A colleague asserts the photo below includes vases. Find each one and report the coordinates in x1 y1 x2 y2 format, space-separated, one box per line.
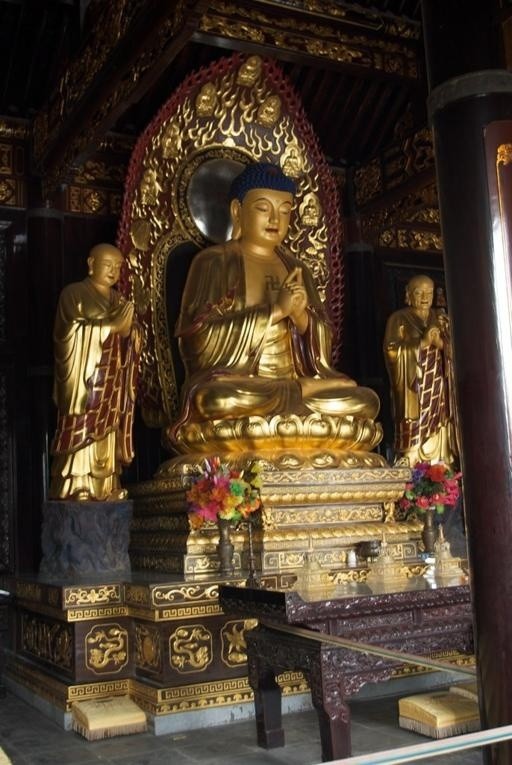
420 506 439 553
215 517 235 573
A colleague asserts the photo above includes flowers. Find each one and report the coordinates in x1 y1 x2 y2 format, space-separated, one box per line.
185 454 265 528
394 462 463 523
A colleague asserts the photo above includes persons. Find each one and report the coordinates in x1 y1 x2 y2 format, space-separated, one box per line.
173 159 386 452
46 243 149 504
382 273 462 471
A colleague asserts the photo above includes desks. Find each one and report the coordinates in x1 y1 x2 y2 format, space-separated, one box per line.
218 558 474 762
